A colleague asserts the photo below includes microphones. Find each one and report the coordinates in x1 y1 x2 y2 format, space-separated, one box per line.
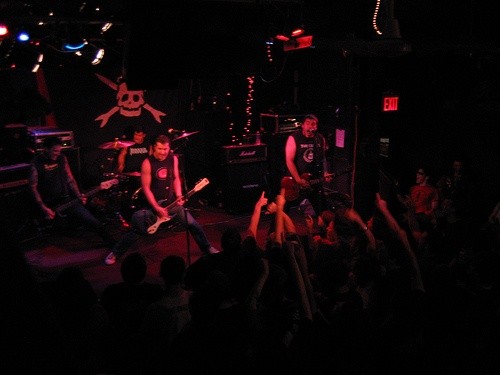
310 128 319 138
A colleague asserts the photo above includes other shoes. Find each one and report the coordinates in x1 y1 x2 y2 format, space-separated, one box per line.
105 251 116 265
201 246 220 254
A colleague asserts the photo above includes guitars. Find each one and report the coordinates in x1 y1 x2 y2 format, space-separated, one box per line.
281 164 359 202
141 177 211 236
34 178 120 237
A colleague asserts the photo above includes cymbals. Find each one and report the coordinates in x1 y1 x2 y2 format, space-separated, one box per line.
97 140 136 150
121 170 141 177
170 129 200 142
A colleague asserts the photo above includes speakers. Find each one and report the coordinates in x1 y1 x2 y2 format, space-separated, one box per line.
0 188 44 243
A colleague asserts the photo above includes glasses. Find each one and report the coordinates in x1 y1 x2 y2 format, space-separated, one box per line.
417 169 428 176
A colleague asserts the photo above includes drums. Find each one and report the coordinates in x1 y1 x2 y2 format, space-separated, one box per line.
130 186 180 231
102 172 128 196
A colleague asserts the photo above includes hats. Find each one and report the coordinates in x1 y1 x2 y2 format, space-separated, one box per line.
305 113 318 122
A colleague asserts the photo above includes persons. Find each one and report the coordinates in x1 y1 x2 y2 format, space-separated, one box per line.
284 113 331 216
15 136 118 248
104 135 221 266
397 166 440 223
0 188 500 375
